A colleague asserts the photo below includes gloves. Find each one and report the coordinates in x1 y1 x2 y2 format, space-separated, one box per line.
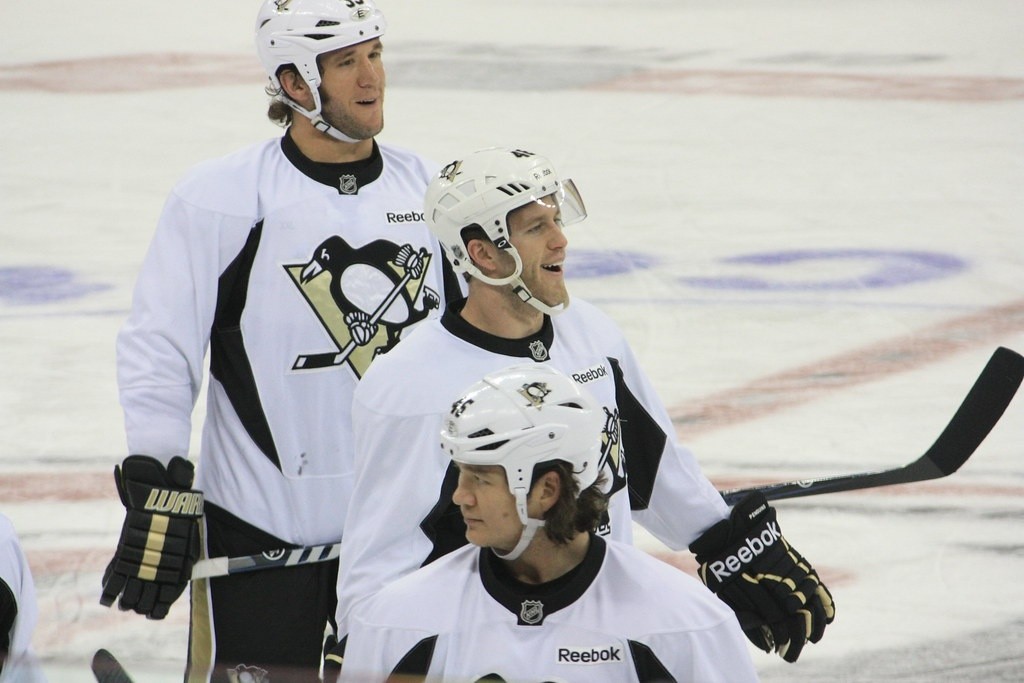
693 492 833 659
101 460 206 616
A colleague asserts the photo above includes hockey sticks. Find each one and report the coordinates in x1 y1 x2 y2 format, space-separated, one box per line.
187 345 1024 585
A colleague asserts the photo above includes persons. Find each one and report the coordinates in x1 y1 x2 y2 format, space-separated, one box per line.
327 365 759 682
0 510 44 683
324 148 839 682
98 2 456 682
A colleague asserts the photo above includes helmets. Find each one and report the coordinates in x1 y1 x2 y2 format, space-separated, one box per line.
256 0 386 142
422 146 587 316
441 361 612 563
